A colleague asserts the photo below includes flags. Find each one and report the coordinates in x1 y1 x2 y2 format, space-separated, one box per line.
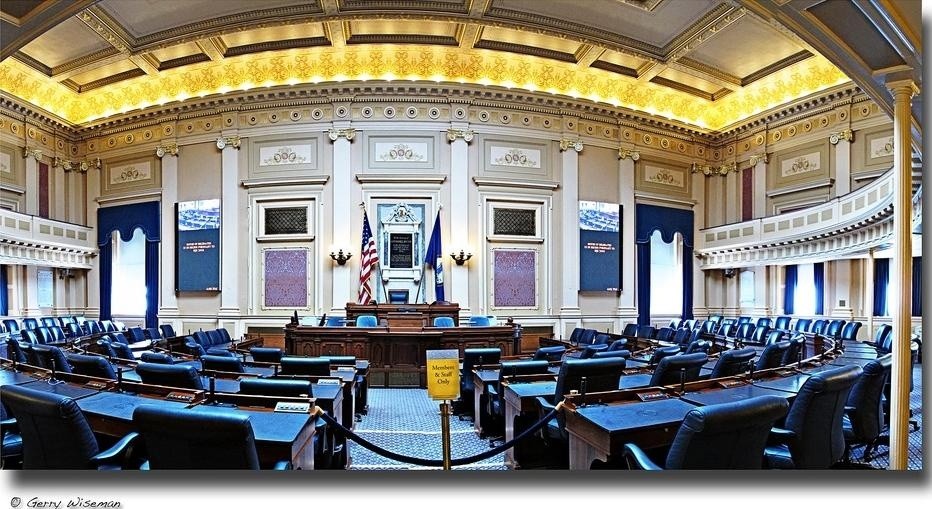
356 206 382 305
426 212 449 303
